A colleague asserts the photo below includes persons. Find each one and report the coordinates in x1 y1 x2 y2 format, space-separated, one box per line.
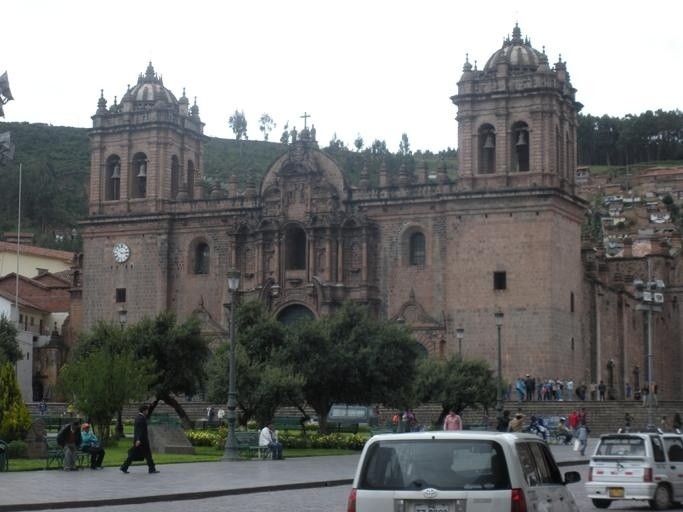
557 407 592 456
669 444 683 462
434 402 463 431
393 408 418 433
575 380 606 401
79 423 105 470
504 375 576 403
56 420 83 471
258 423 285 460
217 407 225 421
617 410 683 434
625 382 659 408
38 399 47 415
496 407 547 440
206 405 216 421
120 405 160 475
67 402 74 417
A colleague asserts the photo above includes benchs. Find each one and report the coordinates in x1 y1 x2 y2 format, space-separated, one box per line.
542 426 570 444
234 431 273 460
43 437 92 469
31 415 81 433
271 416 306 436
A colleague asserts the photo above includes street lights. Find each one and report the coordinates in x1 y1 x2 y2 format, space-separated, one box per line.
633 278 665 430
221 265 244 459
607 359 617 400
631 365 642 401
455 323 464 357
492 309 504 420
0 68 18 162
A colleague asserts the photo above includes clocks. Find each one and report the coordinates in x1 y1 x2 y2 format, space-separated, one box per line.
113 243 131 264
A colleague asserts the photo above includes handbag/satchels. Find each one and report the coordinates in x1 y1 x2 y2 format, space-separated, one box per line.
128 442 145 461
91 441 100 448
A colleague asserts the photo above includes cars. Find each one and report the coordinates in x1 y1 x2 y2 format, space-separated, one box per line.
585 428 682 508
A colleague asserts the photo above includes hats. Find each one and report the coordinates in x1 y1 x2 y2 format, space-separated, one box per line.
82 424 90 429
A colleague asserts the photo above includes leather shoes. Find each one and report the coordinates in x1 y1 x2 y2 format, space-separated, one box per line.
149 470 160 473
120 468 129 473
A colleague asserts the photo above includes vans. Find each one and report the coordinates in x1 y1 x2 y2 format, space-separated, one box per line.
346 431 582 512
327 404 372 426
